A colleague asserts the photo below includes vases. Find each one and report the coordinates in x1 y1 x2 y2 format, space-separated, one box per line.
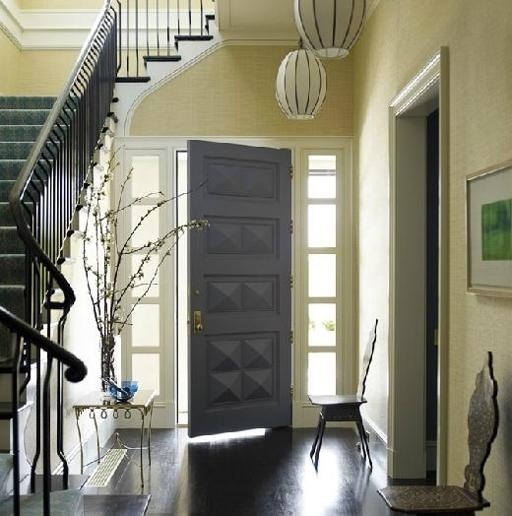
97 339 117 399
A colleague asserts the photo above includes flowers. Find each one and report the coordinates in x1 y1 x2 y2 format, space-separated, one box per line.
81 145 208 393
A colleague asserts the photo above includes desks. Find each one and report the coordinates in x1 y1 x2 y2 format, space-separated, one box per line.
71 392 155 488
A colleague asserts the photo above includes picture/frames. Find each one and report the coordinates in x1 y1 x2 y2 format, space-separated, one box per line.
463 160 512 299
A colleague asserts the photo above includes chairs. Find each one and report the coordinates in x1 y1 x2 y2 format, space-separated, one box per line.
308 319 379 472
377 350 498 516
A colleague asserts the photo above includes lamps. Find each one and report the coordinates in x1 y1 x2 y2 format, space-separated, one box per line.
293 0 368 59
274 36 328 121
